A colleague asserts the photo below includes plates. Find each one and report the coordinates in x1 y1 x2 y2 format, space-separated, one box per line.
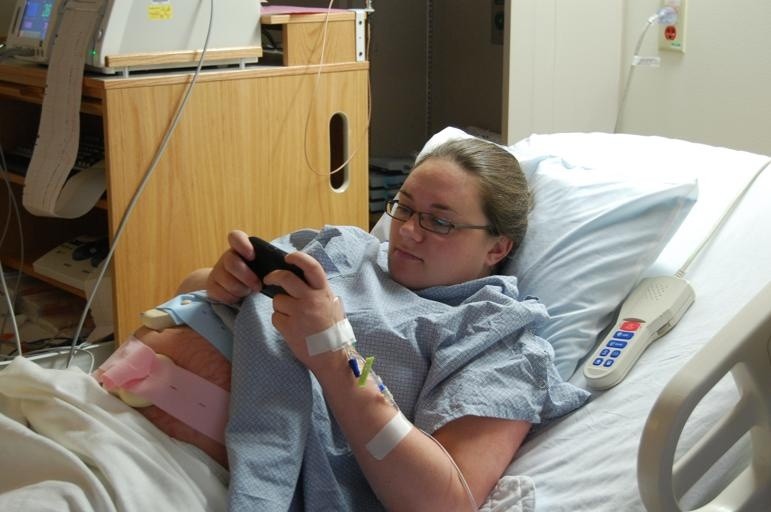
1 10 370 349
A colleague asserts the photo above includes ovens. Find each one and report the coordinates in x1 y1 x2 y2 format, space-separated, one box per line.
0 135 771 511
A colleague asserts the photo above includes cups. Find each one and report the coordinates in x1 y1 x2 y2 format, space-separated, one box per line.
385 199 488 234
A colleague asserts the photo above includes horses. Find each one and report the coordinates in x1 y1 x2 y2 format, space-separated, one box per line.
244 237 304 297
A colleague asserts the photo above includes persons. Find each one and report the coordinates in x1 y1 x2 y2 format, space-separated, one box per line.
0 138 591 512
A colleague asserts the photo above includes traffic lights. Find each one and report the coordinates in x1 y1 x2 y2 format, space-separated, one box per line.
379 127 771 380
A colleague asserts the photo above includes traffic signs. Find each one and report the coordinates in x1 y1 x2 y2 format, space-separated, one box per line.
658 0 686 52
